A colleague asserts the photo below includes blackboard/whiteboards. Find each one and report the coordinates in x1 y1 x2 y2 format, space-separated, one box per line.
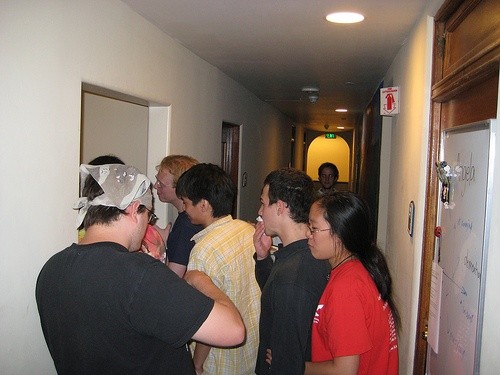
424 118 494 375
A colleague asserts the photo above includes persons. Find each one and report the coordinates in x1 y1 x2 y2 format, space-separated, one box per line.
312 162 339 198
175 163 261 375
266 191 402 375
35 155 246 375
154 153 205 279
253 167 332 375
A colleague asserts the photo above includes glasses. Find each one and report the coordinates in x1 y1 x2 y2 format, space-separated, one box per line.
145 206 159 225
307 225 331 235
154 176 176 188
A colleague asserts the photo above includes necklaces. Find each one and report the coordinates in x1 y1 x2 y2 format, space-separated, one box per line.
325 254 354 281
